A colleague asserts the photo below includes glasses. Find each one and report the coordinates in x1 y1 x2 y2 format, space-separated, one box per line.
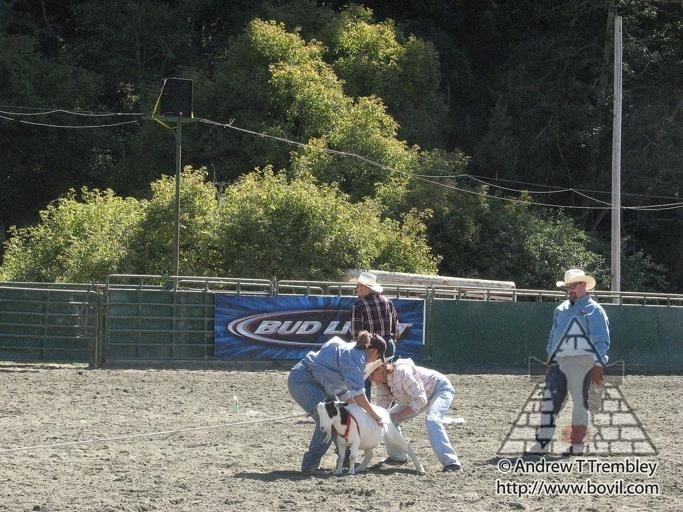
564 282 583 291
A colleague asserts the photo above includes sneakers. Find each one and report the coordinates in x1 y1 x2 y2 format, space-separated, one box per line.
569 443 584 454
525 439 549 453
303 469 333 479
378 457 410 468
442 464 463 476
337 460 361 469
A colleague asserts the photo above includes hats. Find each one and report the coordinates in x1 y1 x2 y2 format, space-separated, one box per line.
363 355 395 381
373 333 386 364
348 272 384 293
555 269 595 291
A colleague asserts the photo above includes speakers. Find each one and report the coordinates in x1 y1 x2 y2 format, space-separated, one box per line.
161 78 193 121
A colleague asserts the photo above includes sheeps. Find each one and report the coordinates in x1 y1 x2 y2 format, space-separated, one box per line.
306 400 425 475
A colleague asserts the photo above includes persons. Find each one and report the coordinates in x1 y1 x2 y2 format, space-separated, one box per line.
363 357 460 473
349 271 400 403
287 329 386 477
527 268 609 455
